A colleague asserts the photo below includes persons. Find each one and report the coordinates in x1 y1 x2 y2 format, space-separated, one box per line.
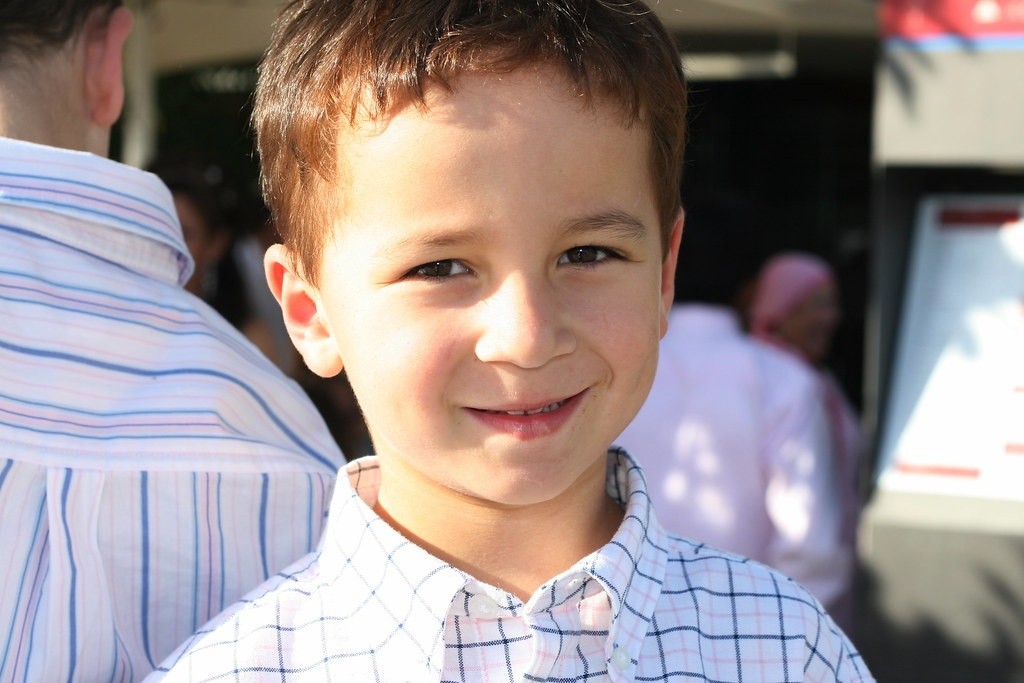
146 0 876 683
0 1 877 683
